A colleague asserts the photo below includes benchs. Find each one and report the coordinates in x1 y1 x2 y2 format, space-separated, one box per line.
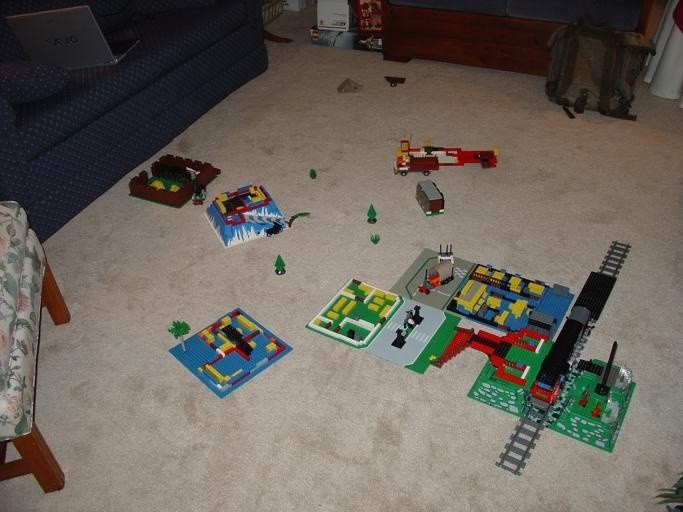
379 1 666 88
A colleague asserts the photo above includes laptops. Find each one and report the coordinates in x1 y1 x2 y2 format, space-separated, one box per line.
6 5 143 72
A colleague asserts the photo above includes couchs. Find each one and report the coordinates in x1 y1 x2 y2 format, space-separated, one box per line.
0 0 268 246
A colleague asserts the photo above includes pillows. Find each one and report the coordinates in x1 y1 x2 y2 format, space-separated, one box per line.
0 58 75 103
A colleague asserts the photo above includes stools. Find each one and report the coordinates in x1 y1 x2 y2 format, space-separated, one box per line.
261 0 295 44
0 199 72 493
